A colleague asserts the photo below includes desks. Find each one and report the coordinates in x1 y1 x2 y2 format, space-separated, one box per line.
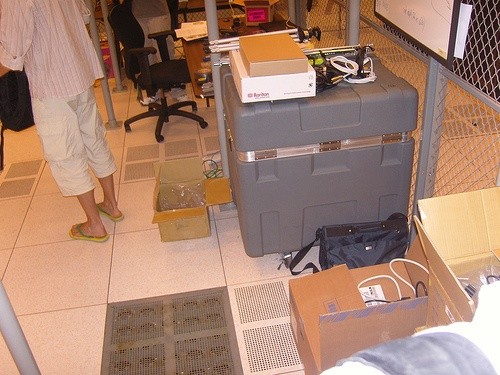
181 23 286 98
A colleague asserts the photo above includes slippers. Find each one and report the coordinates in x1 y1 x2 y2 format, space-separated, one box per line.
69 222 109 242
95 202 124 222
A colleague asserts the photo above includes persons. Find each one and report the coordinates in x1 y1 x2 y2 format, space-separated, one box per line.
131 0 187 105
315 281 500 375
0 0 125 242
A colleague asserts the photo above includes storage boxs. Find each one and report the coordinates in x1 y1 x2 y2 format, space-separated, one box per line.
151 157 231 243
229 49 317 104
239 33 308 76
220 51 419 258
231 0 279 27
414 186 500 322
288 236 428 375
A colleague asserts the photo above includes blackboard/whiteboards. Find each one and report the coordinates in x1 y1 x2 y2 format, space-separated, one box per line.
374 0 461 70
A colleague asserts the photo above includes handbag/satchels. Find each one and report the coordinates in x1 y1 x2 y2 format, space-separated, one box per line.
316 212 410 270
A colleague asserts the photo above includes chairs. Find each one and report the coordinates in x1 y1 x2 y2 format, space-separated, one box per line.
107 2 208 142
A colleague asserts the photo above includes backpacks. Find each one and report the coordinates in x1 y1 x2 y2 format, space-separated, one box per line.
0 68 34 131
